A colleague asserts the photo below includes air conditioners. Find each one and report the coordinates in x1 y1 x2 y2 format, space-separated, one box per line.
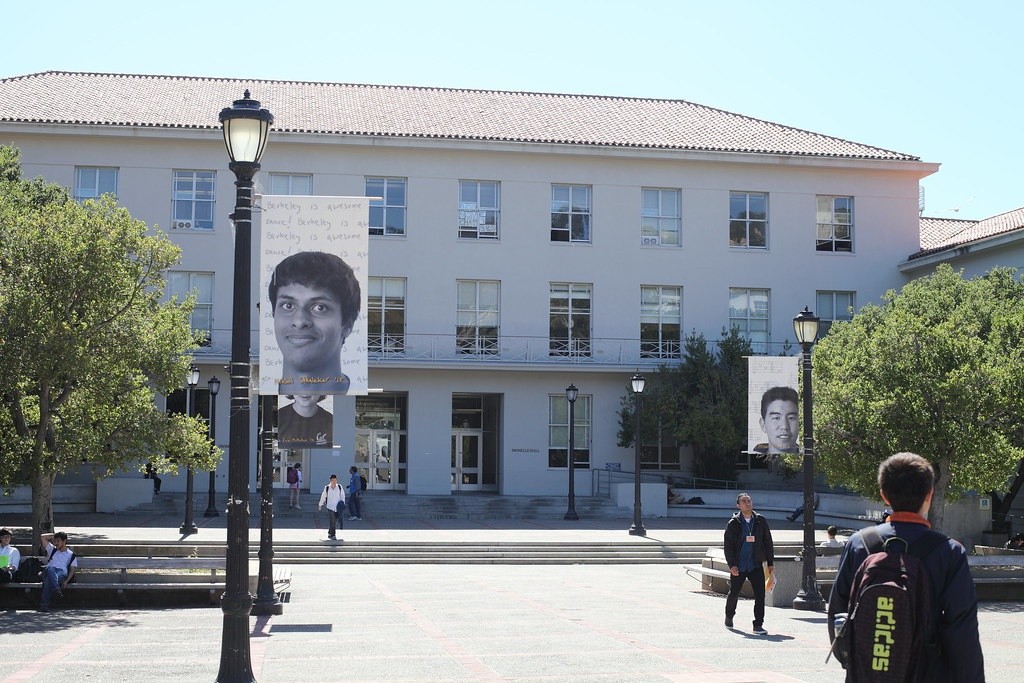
176 220 194 230
640 236 658 246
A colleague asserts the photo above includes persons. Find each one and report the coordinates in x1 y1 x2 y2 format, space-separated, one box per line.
288 462 303 509
36 533 78 612
667 482 677 504
815 525 846 569
317 474 345 541
753 387 798 454
875 512 891 525
723 493 775 634
786 489 820 522
828 451 986 683
272 452 281 482
0 528 21 585
143 463 162 495
346 466 362 521
260 251 366 395
278 395 333 449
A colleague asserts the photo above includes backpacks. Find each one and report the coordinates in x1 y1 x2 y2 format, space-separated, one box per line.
825 527 950 682
18 558 44 583
286 467 298 484
360 474 367 491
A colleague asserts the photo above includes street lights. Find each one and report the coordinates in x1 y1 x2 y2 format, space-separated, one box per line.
203 376 221 517
215 89 274 682
790 305 827 612
628 368 647 536
564 383 579 519
178 365 201 534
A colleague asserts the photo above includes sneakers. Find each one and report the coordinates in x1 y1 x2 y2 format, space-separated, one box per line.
349 516 357 520
328 534 331 538
753 625 768 633
725 616 733 629
330 536 337 541
357 518 362 520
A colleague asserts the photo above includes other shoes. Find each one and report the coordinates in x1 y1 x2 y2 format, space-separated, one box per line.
295 506 301 509
55 590 64 601
37 606 46 612
289 505 292 509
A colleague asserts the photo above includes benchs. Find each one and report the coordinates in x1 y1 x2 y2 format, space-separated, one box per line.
666 487 895 530
0 544 228 605
